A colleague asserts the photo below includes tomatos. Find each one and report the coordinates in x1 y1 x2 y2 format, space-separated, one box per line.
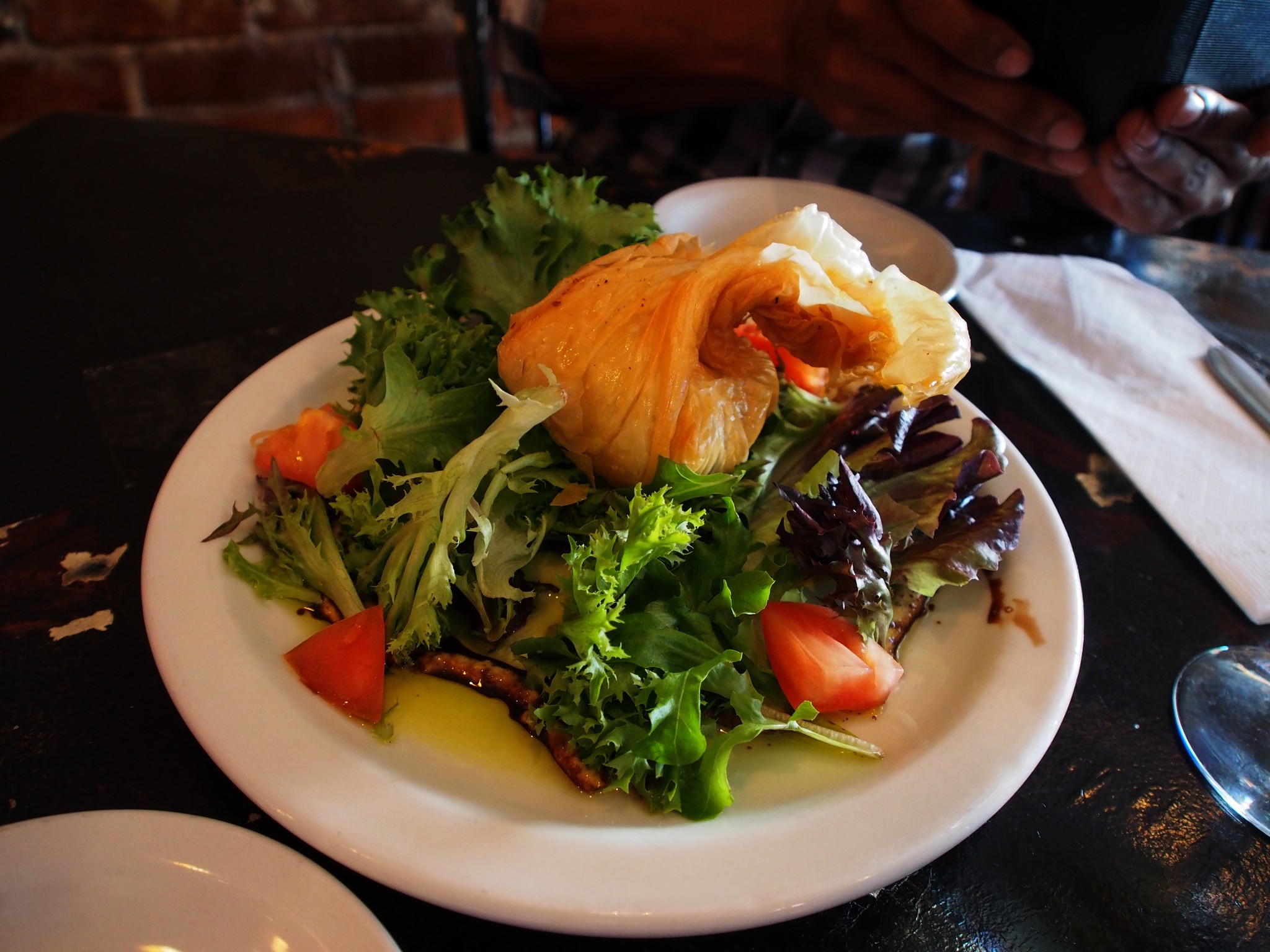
759 598 902 716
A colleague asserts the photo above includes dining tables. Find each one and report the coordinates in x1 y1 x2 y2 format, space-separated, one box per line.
1 106 1270 951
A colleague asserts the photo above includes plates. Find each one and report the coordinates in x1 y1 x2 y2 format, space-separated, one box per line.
142 292 1083 936
0 809 404 952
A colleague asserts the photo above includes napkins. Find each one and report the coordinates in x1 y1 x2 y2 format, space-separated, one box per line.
948 249 1270 626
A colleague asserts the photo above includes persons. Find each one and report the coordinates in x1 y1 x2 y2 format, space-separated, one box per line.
497 0 1269 241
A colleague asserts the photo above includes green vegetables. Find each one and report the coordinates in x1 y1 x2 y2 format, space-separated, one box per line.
203 163 884 821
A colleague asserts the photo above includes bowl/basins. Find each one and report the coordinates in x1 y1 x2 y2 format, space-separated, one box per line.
651 176 960 302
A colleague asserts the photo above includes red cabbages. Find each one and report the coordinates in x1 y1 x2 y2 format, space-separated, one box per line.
746 380 1025 632
